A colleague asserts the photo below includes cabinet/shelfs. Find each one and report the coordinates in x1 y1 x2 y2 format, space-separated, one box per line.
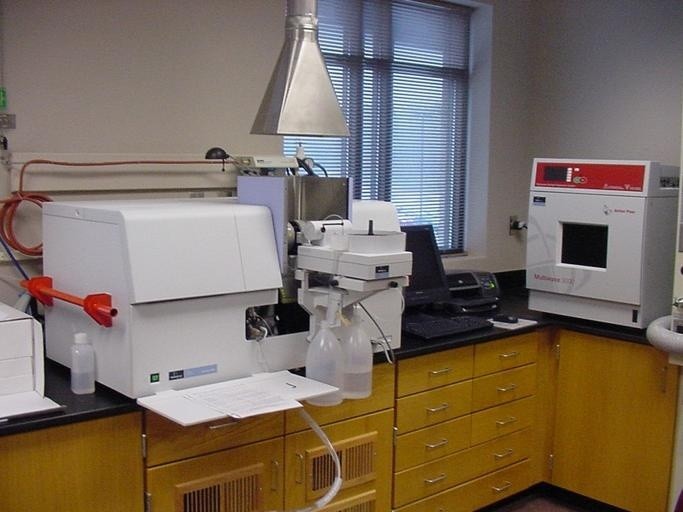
549 329 678 512
395 326 539 512
0 410 145 512
144 362 395 512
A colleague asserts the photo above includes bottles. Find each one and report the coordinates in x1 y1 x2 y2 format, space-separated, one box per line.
71 333 94 394
306 321 344 407
342 316 372 398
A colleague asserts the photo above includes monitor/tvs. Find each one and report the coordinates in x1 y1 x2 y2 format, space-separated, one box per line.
400 224 451 308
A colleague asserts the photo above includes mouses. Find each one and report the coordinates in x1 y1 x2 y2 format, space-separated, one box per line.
493 313 518 324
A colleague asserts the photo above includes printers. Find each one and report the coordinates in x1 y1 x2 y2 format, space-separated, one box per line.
446 268 503 316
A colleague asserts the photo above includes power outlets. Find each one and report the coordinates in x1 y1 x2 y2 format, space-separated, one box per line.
510 215 518 235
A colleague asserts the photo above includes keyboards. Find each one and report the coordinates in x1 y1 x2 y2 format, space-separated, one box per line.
402 313 494 341
